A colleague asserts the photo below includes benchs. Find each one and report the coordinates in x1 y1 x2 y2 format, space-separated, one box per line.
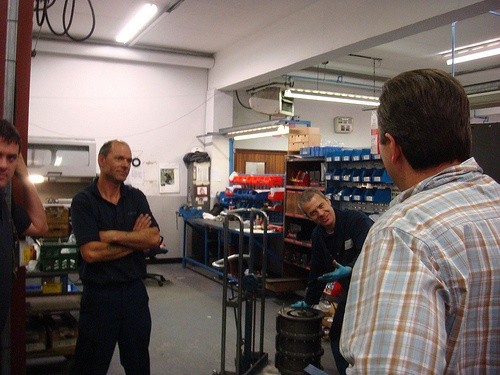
22 293 82 349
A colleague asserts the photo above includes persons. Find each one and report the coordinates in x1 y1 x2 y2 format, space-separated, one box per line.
0 120 48 375
290 188 373 375
70 140 164 375
338 68 500 375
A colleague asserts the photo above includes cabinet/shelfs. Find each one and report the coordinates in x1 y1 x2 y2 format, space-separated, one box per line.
282 157 326 283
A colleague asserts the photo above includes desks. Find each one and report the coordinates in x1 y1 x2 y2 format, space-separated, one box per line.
188 215 283 281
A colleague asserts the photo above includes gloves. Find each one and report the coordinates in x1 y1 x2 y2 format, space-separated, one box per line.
290 301 310 308
318 260 351 282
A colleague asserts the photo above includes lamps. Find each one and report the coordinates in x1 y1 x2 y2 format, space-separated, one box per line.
446 47 500 67
115 3 159 44
282 58 381 106
224 123 290 142
